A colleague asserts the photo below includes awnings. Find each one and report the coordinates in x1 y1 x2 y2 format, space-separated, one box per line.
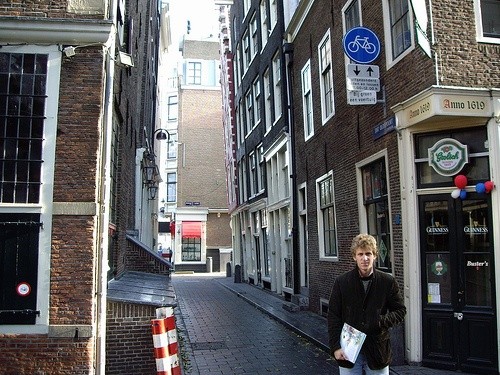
182 221 201 239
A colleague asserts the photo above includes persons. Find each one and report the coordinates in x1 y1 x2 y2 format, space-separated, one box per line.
328 234 406 375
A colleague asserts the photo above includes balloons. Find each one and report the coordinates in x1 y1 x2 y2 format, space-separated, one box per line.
451 189 467 199
455 175 467 189
476 181 493 193
